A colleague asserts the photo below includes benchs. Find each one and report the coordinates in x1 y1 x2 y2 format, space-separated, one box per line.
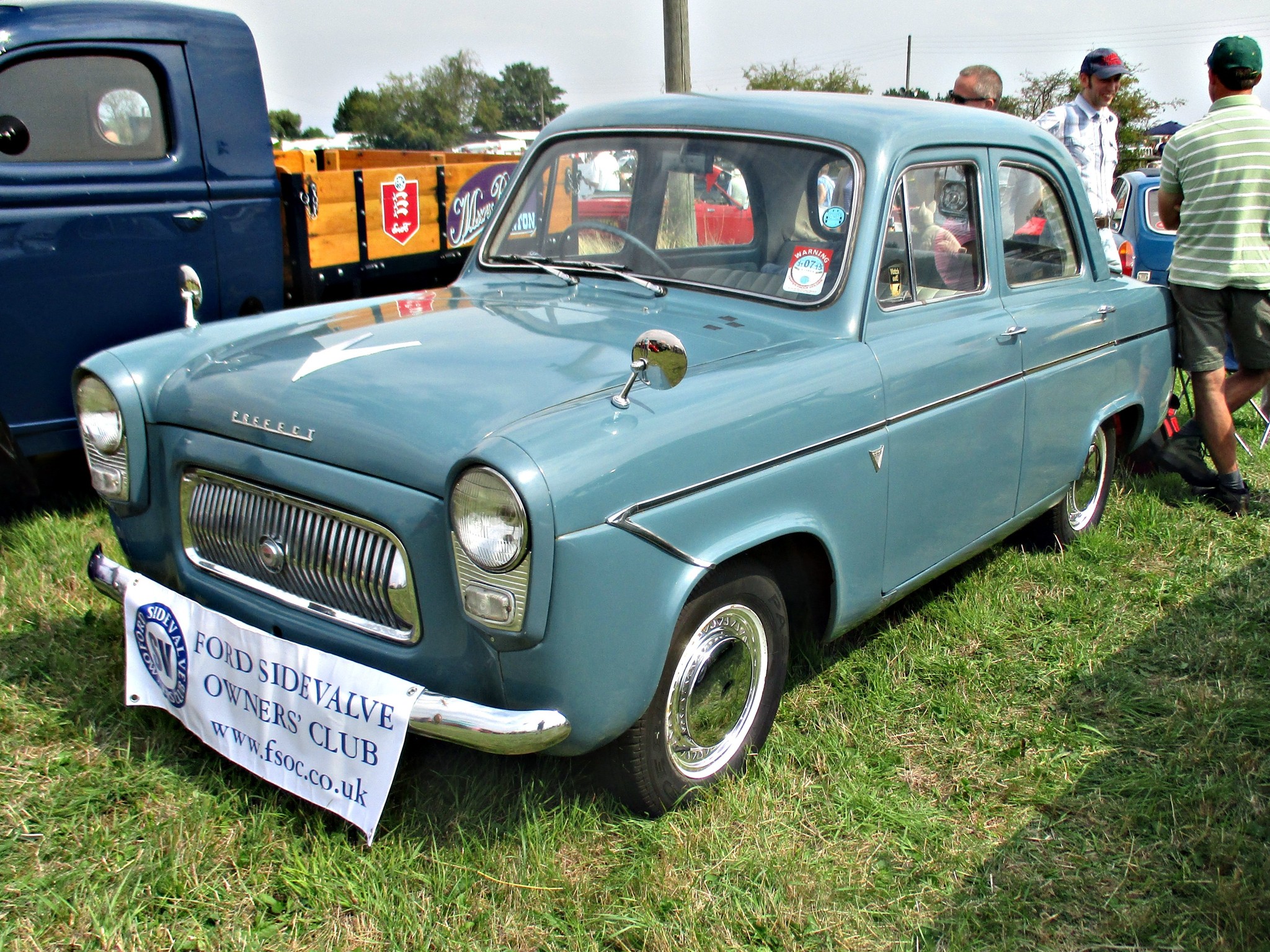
778 241 1064 301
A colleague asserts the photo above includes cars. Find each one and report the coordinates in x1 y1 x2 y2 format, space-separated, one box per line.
1096 168 1180 289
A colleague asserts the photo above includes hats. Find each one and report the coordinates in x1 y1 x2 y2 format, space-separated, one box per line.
1208 35 1262 80
1079 48 1130 78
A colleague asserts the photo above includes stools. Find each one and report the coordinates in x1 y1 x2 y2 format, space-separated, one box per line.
1174 336 1270 455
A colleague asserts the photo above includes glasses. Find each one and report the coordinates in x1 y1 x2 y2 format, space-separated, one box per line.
948 90 997 105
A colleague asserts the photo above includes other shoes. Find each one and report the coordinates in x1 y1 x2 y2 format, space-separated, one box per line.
1192 481 1250 520
1156 432 1219 487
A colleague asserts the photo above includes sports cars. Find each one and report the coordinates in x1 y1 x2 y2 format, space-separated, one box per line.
577 164 754 247
69 89 1180 823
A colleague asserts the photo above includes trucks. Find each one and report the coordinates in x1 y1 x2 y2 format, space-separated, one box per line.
0 1 580 514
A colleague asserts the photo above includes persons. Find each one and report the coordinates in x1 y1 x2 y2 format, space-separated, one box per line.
914 64 1042 241
1158 35 1270 515
818 163 835 228
725 169 749 209
580 151 621 200
829 164 854 233
1031 49 1133 273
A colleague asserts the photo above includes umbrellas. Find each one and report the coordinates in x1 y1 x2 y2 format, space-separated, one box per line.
1142 121 1184 135
